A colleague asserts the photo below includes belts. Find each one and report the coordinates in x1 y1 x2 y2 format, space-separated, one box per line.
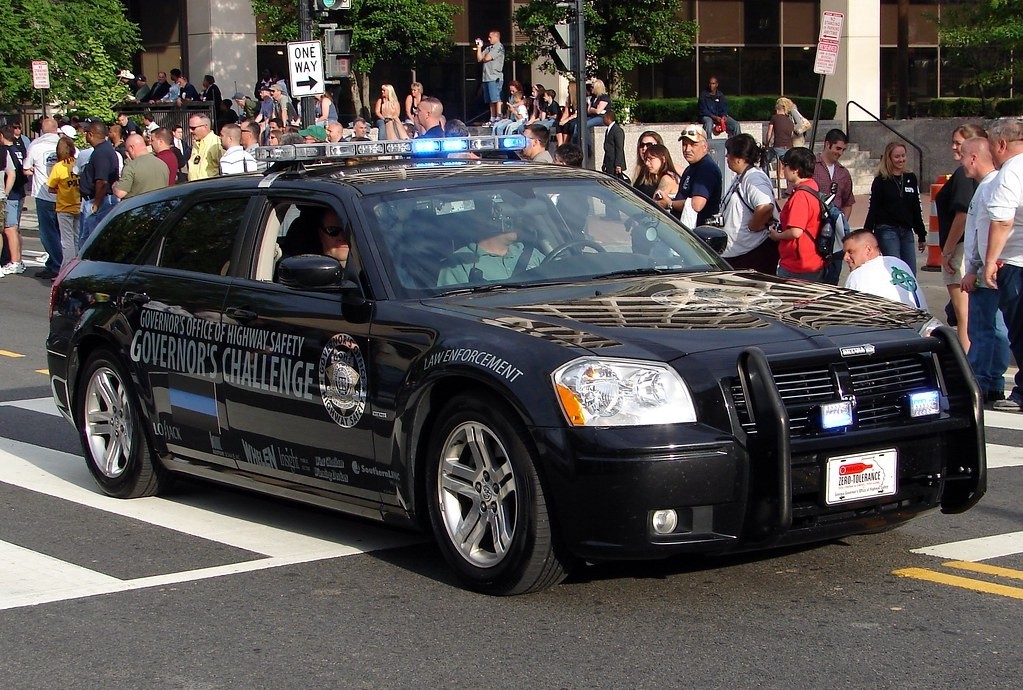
85 191 112 201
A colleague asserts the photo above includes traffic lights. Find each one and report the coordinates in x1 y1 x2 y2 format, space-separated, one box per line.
321 32 354 79
314 0 344 10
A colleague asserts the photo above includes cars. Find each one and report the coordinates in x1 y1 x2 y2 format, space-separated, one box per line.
44 133 989 596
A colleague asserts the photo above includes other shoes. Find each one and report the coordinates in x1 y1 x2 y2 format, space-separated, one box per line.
36 252 50 263
36 269 57 279
993 397 1021 410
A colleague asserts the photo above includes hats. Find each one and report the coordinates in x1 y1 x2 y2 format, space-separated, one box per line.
257 85 268 91
117 69 135 79
678 124 708 142
57 125 78 141
298 126 327 142
233 93 245 99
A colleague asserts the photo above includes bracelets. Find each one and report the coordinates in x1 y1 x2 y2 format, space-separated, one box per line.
941 252 951 258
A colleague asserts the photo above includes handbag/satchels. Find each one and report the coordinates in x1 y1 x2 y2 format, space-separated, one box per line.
614 171 632 185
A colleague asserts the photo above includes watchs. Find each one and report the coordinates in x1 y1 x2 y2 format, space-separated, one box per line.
668 200 673 211
384 117 392 124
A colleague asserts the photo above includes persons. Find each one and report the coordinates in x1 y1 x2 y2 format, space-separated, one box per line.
864 141 928 279
837 229 929 317
935 123 996 356
976 118 1023 411
475 30 505 128
436 192 556 286
316 207 416 292
0 68 855 282
960 137 1011 404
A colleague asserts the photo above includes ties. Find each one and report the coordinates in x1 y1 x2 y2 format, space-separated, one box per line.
178 140 181 150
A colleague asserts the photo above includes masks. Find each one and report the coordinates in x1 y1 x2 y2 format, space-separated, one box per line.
268 85 281 91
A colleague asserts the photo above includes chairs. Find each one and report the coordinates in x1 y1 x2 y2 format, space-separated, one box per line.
284 216 323 254
428 203 480 284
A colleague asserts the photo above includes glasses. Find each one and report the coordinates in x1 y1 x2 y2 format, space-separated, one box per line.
321 225 343 236
680 130 706 139
590 86 594 89
638 141 653 149
188 125 205 131
13 126 21 129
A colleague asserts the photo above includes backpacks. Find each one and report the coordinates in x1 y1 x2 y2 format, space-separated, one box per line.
790 186 849 260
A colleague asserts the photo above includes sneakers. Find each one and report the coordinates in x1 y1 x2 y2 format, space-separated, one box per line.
2 261 25 275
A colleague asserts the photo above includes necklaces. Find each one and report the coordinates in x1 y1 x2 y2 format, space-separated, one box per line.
893 173 904 198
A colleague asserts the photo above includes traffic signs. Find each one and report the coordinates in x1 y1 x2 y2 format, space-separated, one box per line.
288 40 325 97
